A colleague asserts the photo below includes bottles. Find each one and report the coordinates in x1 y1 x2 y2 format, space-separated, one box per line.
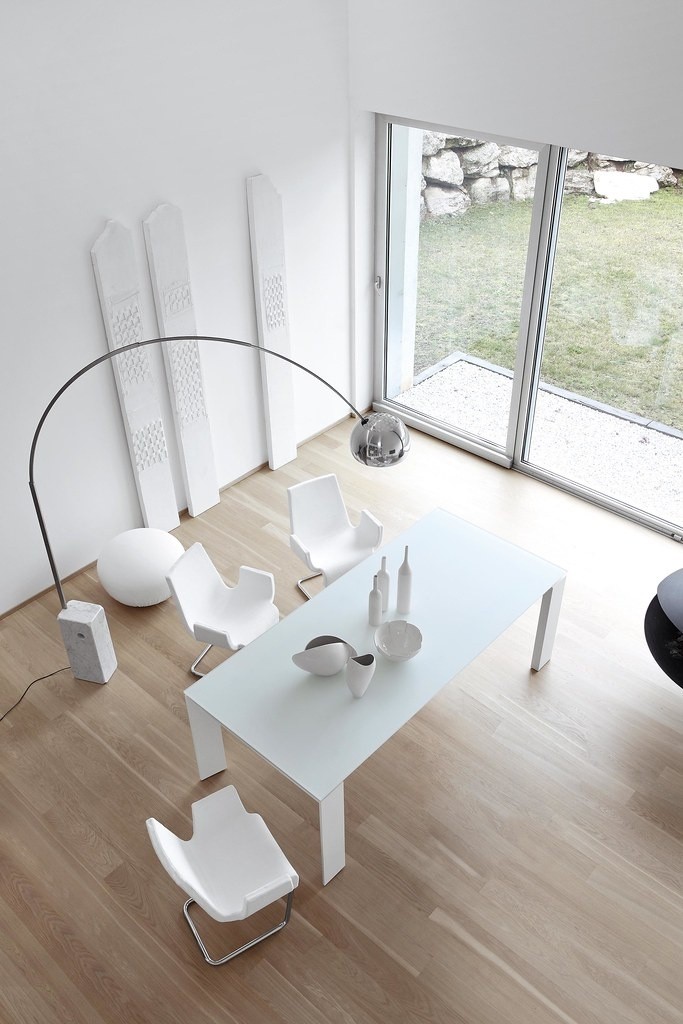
368 575 382 627
396 545 412 614
376 557 390 612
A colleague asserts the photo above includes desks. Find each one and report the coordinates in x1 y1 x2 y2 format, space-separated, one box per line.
183 507 569 886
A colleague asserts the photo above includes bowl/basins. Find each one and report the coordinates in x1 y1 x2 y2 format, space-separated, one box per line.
373 619 423 663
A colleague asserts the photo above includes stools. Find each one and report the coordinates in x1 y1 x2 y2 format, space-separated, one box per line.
97 528 184 606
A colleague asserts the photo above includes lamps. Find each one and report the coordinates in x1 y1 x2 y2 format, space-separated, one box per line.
28 337 410 684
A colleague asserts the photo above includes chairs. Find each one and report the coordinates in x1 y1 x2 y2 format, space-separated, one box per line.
287 474 384 599
166 542 279 677
146 785 299 966
